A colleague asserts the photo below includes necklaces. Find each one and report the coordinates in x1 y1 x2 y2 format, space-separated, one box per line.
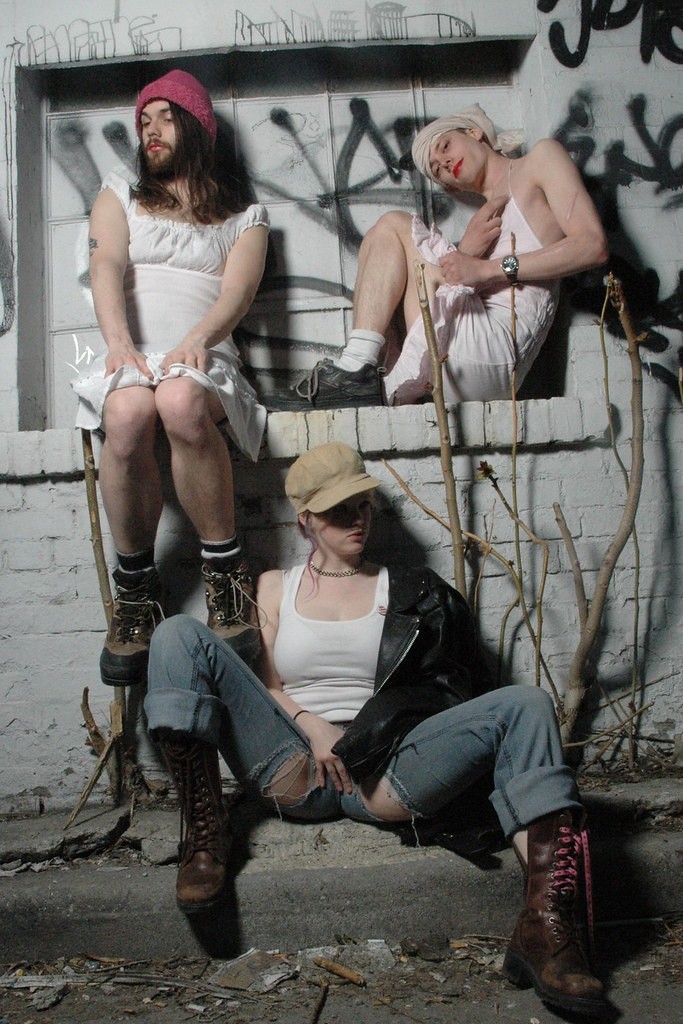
310 554 366 577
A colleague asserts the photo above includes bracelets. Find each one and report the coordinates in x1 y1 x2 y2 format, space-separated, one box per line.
294 710 309 721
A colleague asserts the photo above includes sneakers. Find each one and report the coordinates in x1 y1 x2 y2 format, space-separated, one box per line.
99 569 161 686
200 558 268 663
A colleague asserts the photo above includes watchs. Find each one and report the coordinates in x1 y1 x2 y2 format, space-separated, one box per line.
500 255 519 287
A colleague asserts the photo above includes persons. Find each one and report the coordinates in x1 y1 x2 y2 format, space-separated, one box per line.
68 70 270 686
145 443 611 1018
257 102 609 412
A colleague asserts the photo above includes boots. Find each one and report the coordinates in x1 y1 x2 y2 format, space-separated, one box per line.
501 804 608 1016
159 733 233 911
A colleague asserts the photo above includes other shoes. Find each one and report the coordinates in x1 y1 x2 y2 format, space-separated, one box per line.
262 357 388 411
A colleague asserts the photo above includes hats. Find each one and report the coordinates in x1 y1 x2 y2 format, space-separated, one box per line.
135 69 216 150
284 441 381 514
